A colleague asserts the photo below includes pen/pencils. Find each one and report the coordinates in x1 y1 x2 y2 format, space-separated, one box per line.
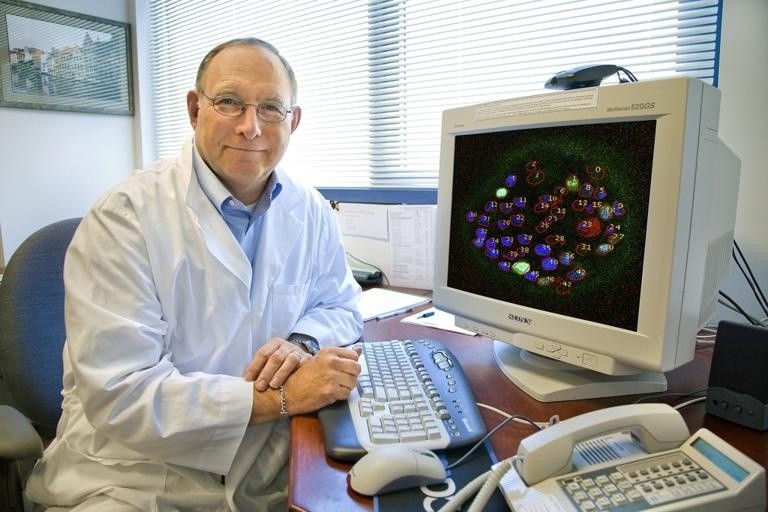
377 307 412 321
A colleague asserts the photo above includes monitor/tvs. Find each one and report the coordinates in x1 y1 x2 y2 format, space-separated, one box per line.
432 74 742 403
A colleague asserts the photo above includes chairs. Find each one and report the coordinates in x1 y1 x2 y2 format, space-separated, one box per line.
0 215 81 511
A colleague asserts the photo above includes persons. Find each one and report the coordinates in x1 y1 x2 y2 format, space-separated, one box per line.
23 37 366 512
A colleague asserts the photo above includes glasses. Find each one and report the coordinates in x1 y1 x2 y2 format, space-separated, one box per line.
202 90 292 123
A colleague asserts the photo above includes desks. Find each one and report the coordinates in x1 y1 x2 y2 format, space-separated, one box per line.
283 285 768 512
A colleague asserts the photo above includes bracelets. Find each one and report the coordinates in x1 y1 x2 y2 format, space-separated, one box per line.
279 385 288 421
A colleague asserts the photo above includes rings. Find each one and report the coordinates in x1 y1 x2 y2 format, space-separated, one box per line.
294 351 301 361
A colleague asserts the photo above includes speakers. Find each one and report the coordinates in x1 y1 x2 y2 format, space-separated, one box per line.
704 321 768 431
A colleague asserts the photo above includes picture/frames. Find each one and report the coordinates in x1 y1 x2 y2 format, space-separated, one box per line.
1 0 137 118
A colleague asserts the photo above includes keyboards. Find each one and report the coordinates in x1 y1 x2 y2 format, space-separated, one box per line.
317 338 489 465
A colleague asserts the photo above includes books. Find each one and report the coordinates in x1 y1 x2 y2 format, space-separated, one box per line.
361 287 431 322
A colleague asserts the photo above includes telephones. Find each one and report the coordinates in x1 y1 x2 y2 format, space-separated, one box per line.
491 403 767 512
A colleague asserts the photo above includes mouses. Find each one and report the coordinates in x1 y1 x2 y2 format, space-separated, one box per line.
344 444 447 496
